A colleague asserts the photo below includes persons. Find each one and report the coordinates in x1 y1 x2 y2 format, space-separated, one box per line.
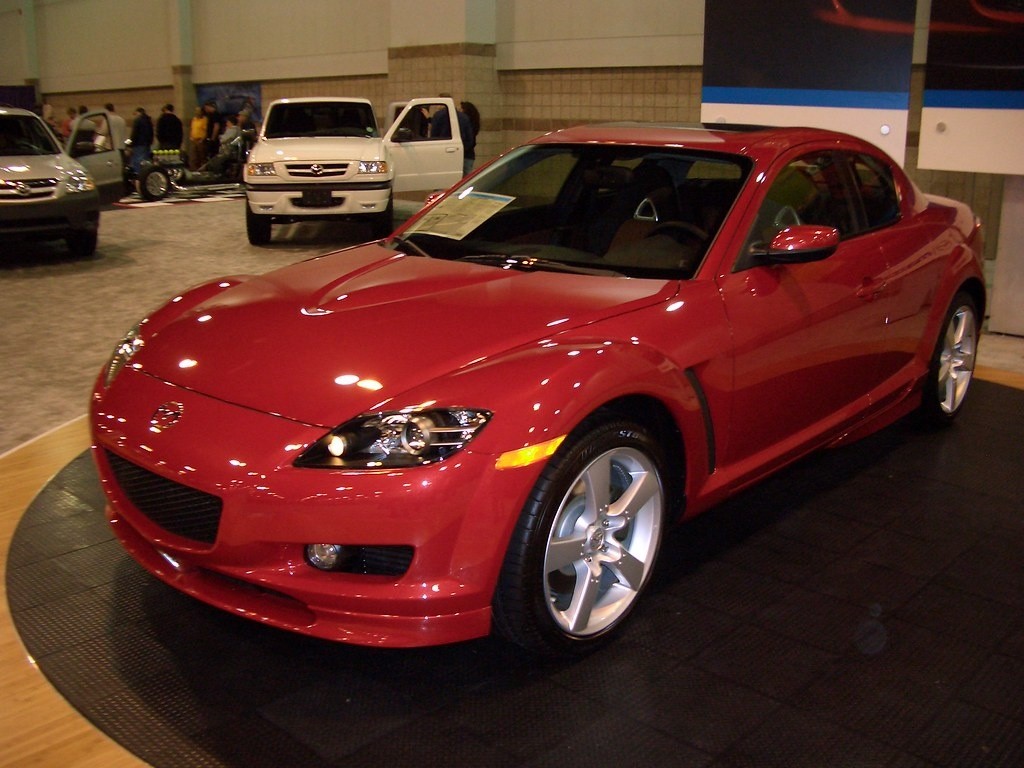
40 104 127 161
422 92 479 180
129 107 154 197
155 98 260 163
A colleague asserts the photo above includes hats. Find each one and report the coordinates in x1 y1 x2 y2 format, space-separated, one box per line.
161 104 173 112
239 111 249 116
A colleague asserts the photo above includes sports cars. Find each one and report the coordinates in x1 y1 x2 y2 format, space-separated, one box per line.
82 120 991 664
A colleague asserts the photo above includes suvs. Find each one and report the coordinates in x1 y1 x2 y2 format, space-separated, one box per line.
236 96 465 245
0 103 124 259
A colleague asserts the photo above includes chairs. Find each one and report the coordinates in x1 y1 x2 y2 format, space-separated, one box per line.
282 108 316 134
340 109 360 128
607 187 700 270
